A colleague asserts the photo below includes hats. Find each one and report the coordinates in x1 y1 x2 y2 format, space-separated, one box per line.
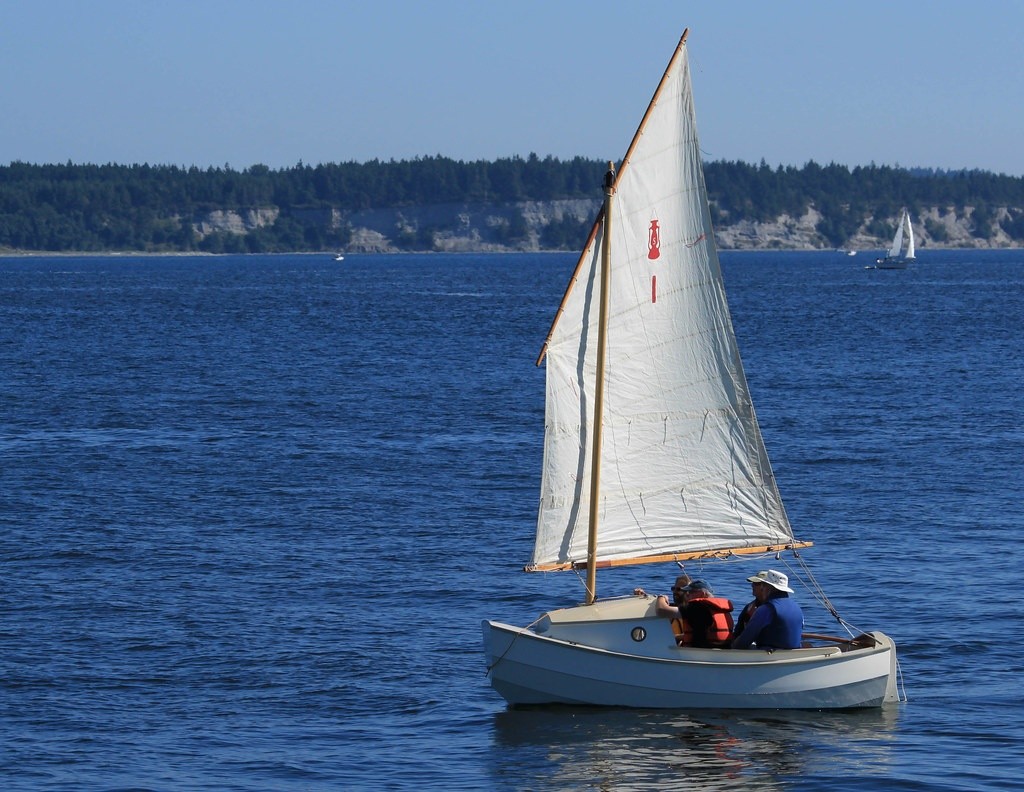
746 571 768 582
681 579 713 592
758 570 795 594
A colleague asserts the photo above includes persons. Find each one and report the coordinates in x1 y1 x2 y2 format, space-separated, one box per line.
633 569 806 650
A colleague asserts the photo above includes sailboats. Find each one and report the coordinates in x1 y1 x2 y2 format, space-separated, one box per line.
876 208 916 269
482 27 908 709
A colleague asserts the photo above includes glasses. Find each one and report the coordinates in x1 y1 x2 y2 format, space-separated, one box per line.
670 586 682 591
752 581 762 586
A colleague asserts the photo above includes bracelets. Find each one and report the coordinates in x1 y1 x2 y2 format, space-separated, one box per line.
657 594 666 600
